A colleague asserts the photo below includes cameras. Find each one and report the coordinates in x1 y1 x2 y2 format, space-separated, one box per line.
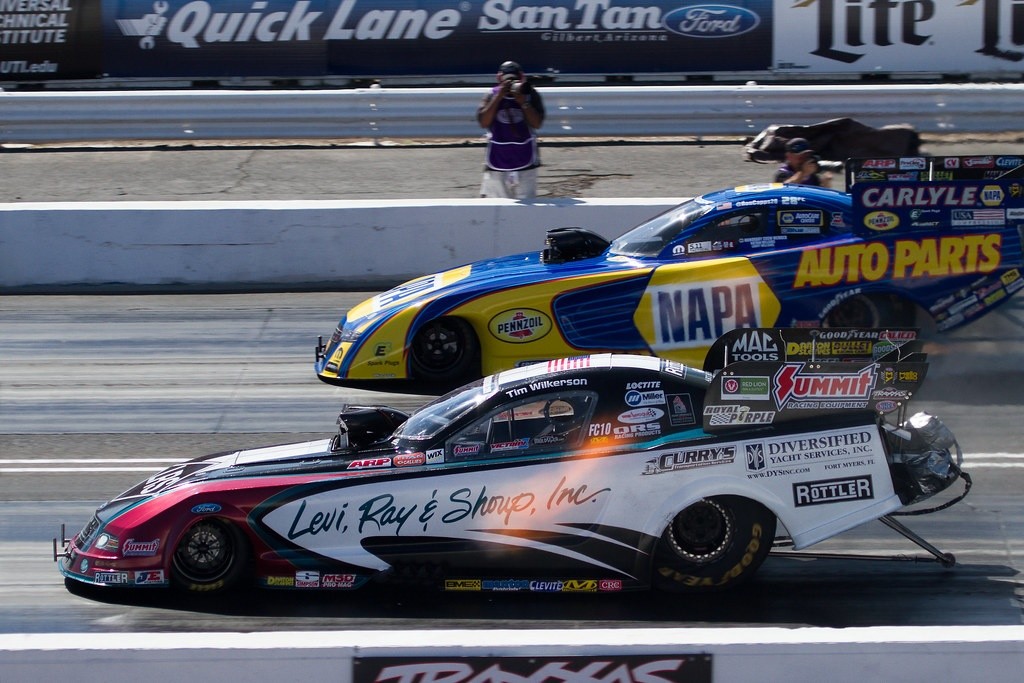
810 155 822 174
507 79 530 94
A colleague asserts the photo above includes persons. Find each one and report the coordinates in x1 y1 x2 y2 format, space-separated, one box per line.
775 138 833 186
476 61 547 198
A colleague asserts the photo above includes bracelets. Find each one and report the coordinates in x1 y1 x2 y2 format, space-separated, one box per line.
522 102 530 109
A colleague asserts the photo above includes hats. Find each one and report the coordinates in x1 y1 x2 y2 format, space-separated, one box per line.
501 61 523 75
785 138 810 153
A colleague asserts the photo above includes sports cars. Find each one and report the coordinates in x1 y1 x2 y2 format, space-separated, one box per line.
312 152 1024 395
54 325 972 615
